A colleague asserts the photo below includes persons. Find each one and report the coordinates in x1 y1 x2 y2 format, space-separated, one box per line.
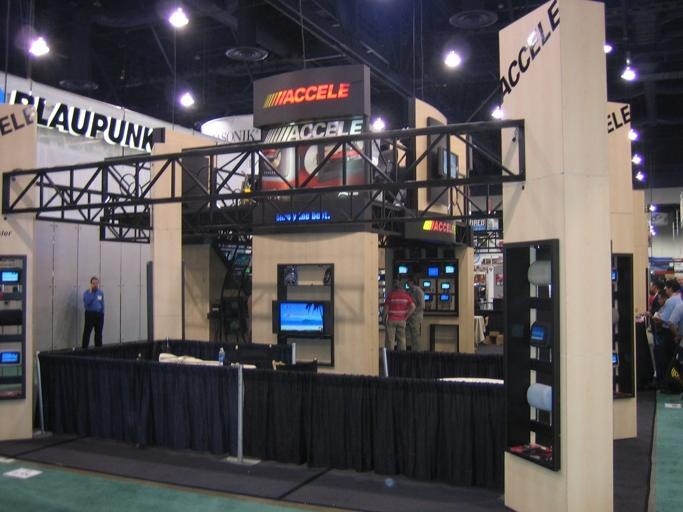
405 273 425 353
383 278 416 351
650 272 683 395
82 276 104 346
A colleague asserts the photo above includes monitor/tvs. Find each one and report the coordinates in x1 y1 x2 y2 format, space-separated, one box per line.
438 146 460 180
272 299 333 337
